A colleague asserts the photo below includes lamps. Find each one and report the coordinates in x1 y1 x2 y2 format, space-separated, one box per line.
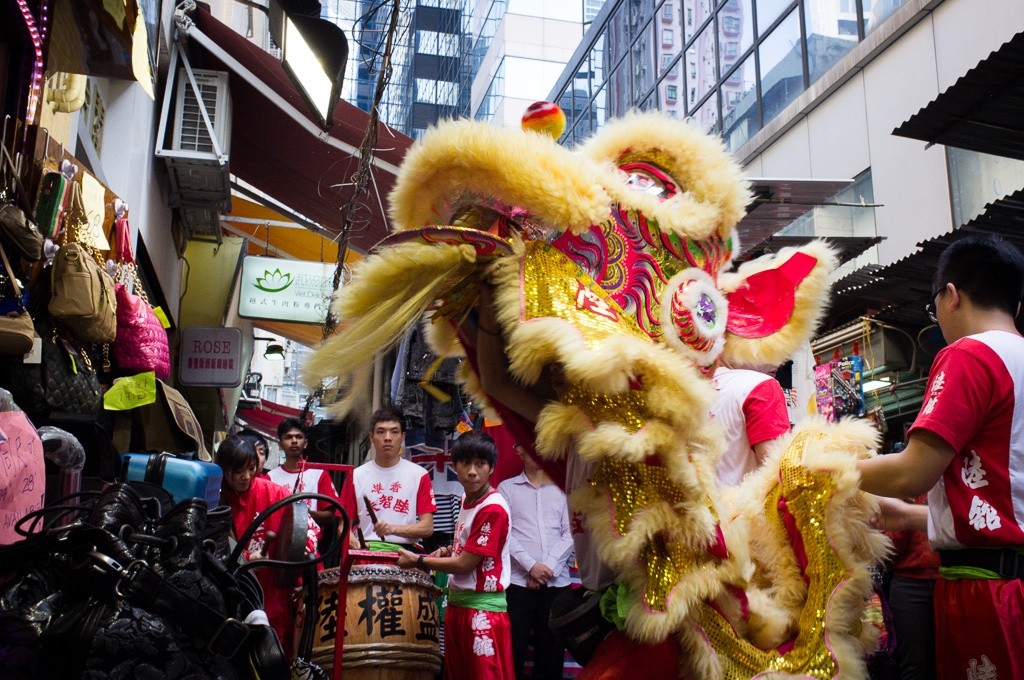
254 337 285 361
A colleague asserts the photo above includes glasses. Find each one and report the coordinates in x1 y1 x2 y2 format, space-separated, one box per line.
925 283 958 325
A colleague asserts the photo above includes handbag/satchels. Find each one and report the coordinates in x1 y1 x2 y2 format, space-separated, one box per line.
1 144 216 464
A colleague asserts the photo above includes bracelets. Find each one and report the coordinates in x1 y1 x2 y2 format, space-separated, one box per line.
444 544 451 555
438 546 443 556
415 554 427 569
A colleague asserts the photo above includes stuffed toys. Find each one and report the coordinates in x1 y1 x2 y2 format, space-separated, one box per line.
298 101 896 680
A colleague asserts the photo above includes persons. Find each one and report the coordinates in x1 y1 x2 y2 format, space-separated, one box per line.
397 429 515 680
496 445 575 680
856 232 1024 680
334 405 437 578
264 415 360 666
215 429 320 651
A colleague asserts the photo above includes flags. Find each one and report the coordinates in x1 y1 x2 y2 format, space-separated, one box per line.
406 428 466 497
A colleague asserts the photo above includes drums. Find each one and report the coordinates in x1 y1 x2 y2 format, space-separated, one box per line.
287 564 445 680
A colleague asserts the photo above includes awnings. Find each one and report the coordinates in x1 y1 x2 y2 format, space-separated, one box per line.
175 0 416 256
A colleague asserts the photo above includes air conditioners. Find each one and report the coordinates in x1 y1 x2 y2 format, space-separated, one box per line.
173 68 232 191
